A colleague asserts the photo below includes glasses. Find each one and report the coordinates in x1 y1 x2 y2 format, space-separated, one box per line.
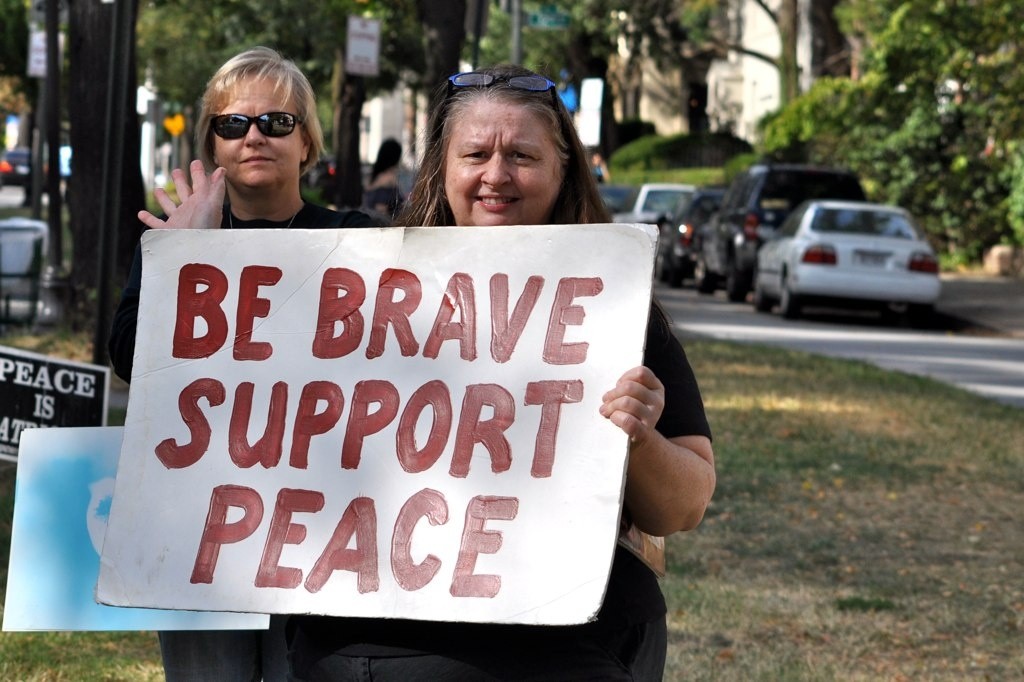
447 72 556 98
209 111 304 141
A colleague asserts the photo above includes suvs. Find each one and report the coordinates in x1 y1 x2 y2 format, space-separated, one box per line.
698 161 869 301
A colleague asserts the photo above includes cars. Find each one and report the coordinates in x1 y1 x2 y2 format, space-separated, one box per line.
1 151 33 187
598 188 635 220
635 182 697 233
653 189 729 285
763 200 942 322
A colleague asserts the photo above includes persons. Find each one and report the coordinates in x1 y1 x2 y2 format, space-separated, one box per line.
108 49 385 682
284 65 718 682
